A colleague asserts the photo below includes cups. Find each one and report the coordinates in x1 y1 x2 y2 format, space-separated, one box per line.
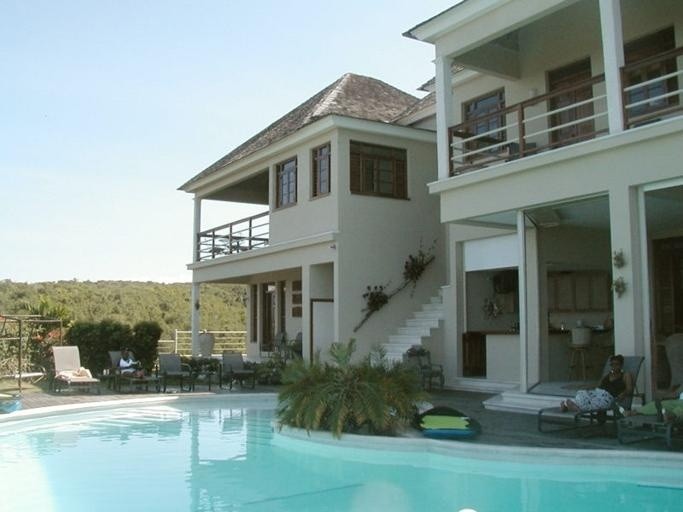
576 319 585 328
559 319 568 330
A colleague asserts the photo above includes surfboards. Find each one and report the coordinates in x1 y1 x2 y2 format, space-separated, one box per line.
415 405 482 440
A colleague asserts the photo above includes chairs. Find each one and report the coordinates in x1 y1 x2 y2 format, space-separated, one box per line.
50 344 260 394
538 353 643 442
407 347 445 395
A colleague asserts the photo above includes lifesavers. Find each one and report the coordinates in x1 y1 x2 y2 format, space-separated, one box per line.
1 399 21 413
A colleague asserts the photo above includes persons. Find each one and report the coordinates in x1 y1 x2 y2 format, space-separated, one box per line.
115 348 144 379
617 384 683 422
560 354 632 413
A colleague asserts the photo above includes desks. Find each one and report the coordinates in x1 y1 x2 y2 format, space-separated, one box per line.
614 414 675 449
